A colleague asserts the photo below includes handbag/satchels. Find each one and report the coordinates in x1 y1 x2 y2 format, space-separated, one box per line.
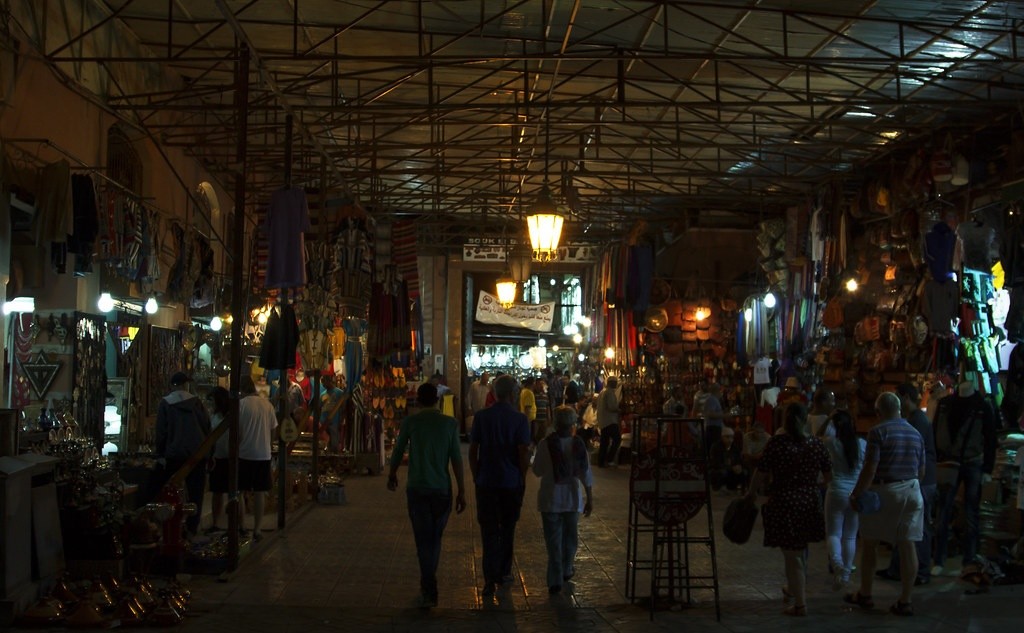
662 269 737 357
818 132 970 434
934 463 961 498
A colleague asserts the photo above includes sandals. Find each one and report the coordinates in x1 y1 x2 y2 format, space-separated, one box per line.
890 600 914 616
784 604 807 616
783 585 794 603
843 592 873 609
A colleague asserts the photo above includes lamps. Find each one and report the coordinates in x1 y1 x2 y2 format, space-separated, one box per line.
526 102 567 257
496 236 516 309
507 179 534 283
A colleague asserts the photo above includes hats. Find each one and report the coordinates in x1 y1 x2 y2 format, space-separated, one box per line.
171 372 190 385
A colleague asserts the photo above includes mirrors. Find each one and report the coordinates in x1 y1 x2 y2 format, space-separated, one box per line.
101 377 132 454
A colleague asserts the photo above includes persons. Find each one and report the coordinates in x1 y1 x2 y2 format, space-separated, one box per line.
662 381 771 490
552 369 578 407
597 377 624 468
842 392 927 618
388 383 467 608
469 376 530 595
206 387 231 536
466 371 536 437
932 381 995 575
579 399 599 439
817 409 867 585
275 377 304 428
747 401 832 617
804 388 835 437
237 375 279 544
158 371 214 537
874 382 936 586
530 405 592 595
320 376 343 452
431 374 450 399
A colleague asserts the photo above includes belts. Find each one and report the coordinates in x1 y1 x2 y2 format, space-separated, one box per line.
884 479 900 484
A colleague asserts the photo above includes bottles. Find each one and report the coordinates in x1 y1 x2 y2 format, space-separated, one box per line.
37 407 78 452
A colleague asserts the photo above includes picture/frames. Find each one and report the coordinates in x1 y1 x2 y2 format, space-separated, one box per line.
147 324 184 417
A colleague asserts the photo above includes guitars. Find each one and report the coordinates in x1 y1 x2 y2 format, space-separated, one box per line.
278 389 298 443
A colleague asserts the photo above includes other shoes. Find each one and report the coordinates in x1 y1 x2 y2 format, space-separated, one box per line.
549 584 561 594
207 526 220 533
915 575 929 584
483 582 495 597
563 570 574 578
238 528 246 535
253 534 264 543
832 567 850 591
416 592 436 608
495 576 513 582
877 569 899 581
